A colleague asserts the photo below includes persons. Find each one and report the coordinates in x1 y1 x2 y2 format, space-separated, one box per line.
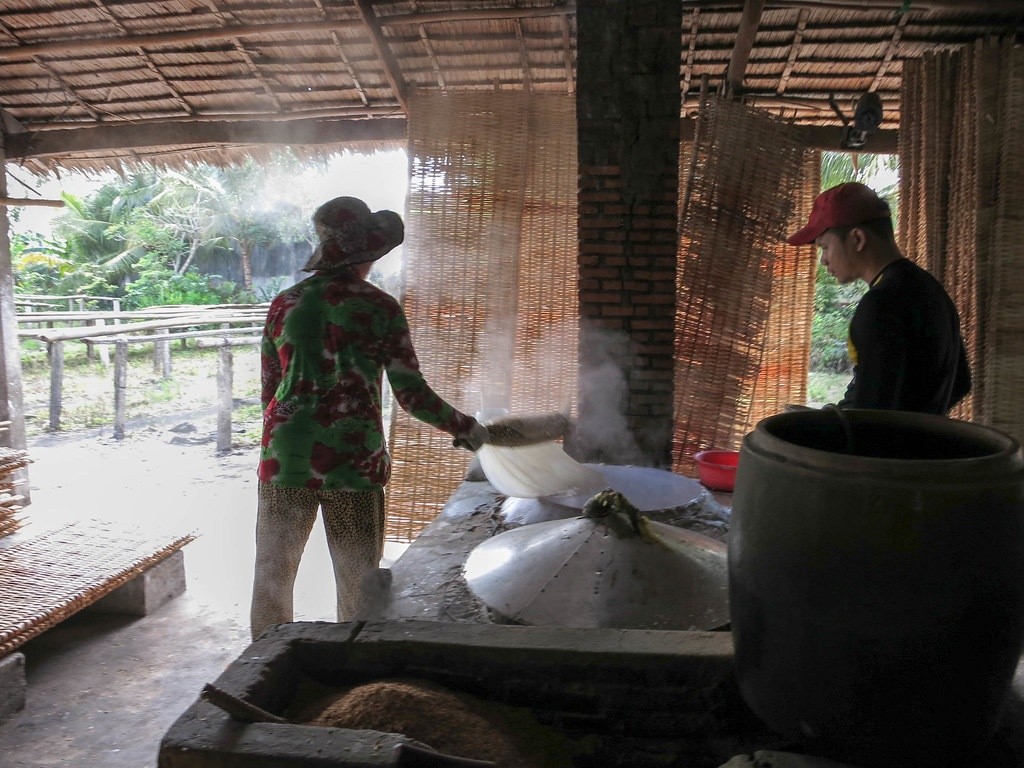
787 181 972 414
250 196 489 640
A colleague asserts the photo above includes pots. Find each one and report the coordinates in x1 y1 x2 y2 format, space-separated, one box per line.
536 462 706 520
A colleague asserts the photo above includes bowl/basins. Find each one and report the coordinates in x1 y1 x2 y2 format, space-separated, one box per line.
693 450 743 493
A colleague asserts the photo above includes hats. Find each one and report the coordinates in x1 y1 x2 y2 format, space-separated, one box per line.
302 196 404 272
787 181 892 245
